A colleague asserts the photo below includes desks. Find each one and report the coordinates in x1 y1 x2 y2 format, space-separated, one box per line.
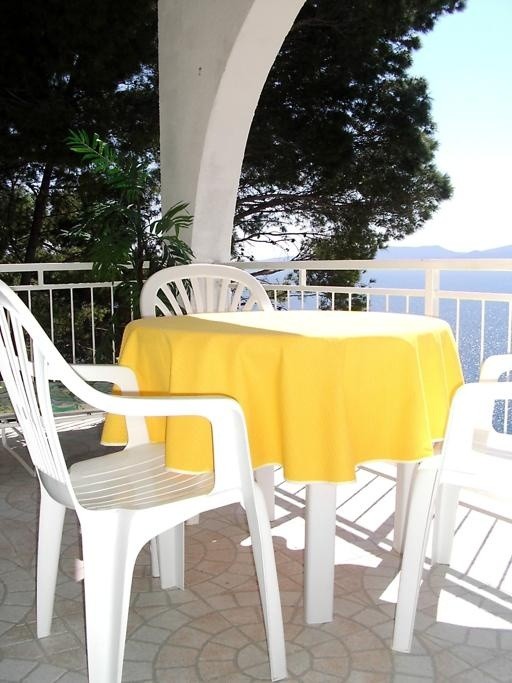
104 310 467 629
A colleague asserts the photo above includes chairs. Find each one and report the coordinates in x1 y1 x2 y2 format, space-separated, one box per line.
138 259 279 525
392 349 512 661
0 278 294 683
